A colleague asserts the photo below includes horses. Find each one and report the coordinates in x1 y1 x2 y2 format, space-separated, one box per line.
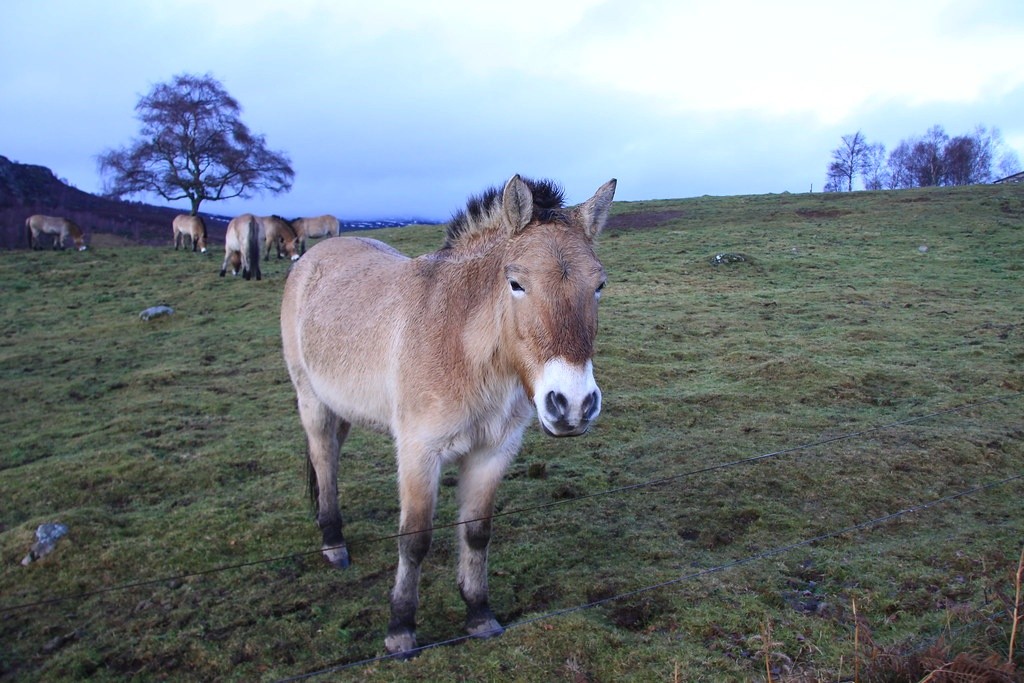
171 214 208 254
218 211 339 283
24 215 87 252
279 172 618 660
